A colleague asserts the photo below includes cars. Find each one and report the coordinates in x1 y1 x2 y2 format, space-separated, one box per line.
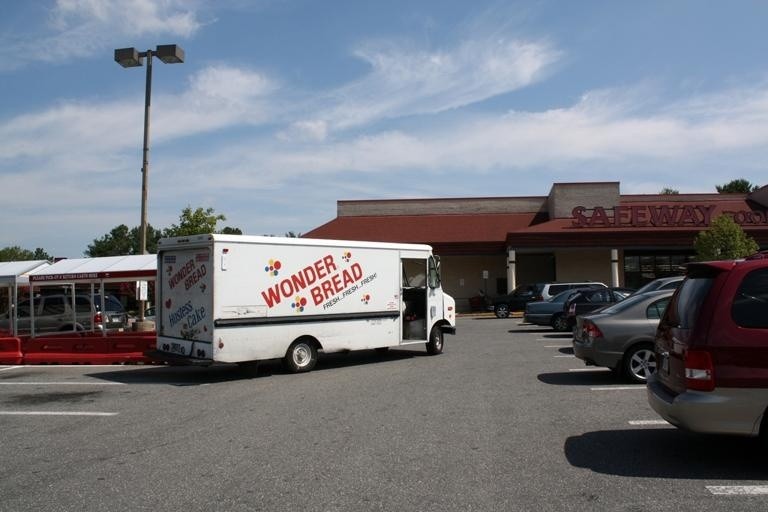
0 291 155 338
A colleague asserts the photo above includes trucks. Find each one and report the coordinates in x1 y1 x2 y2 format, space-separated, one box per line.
156 233 457 374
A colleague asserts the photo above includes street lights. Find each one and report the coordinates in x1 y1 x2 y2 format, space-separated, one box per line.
114 44 185 322
